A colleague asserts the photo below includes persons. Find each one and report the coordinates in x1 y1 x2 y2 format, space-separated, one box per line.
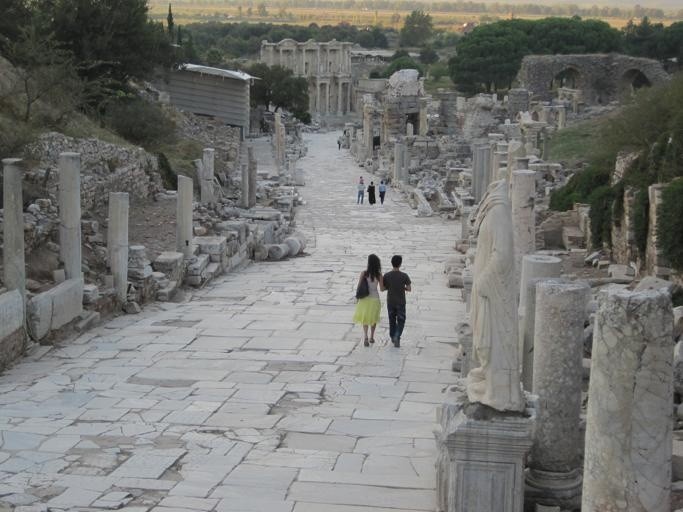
336 136 342 151
383 255 411 346
366 181 376 205
359 176 364 184
353 253 387 347
342 126 346 136
357 183 365 204
378 181 386 206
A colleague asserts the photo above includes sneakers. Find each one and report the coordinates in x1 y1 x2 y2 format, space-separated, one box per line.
363 336 400 347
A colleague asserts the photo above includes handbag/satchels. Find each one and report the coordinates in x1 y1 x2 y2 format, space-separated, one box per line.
355 276 371 299
